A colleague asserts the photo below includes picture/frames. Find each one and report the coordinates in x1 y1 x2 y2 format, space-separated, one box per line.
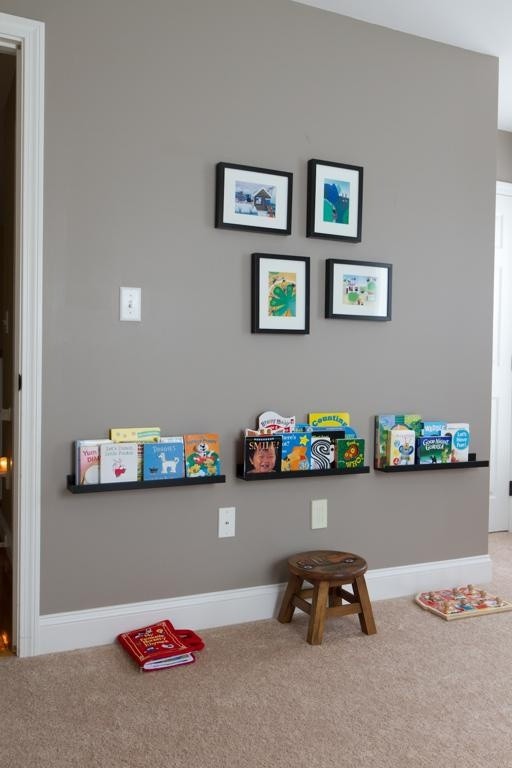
215 158 393 334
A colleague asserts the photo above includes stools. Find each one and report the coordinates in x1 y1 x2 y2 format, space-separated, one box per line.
277 550 376 645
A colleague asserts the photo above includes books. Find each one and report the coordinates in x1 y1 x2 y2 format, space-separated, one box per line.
375 415 469 468
242 412 365 474
74 427 223 485
117 617 198 674
